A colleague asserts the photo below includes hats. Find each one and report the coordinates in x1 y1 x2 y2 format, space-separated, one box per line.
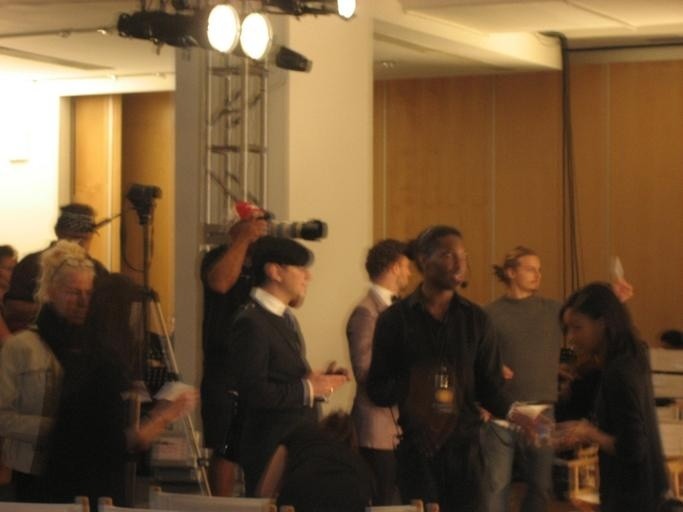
220 200 275 237
250 235 313 267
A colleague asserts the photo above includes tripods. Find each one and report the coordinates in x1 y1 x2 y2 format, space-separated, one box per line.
116 219 211 507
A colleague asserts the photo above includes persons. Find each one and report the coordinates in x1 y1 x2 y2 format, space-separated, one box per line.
346 238 413 506
0 244 17 348
195 200 275 448
0 202 99 336
476 244 563 406
363 220 517 511
42 271 201 511
225 236 341 510
0 238 97 510
517 282 683 512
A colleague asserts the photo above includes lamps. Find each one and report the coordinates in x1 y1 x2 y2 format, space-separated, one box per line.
116 2 356 61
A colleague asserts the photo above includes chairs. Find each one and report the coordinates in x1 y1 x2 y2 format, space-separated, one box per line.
568 415 682 508
0 485 438 511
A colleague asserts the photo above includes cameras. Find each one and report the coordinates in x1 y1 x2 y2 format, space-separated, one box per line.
256 214 323 241
